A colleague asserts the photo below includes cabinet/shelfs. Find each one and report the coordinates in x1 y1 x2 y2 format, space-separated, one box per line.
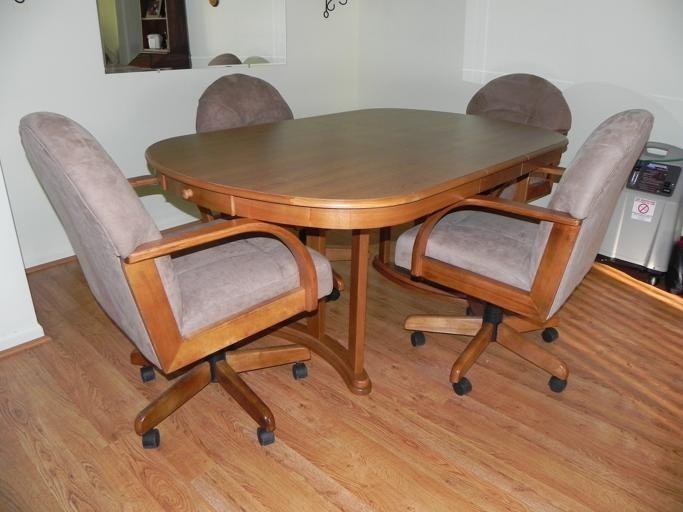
128 0 189 69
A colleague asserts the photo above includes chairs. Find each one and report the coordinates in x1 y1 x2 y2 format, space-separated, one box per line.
373 74 573 308
208 53 270 65
194 73 372 300
397 107 653 395
19 112 342 450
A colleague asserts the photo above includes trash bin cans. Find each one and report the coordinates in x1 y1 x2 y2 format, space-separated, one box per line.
593 142 683 286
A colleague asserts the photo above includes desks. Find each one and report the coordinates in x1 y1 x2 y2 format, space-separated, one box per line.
144 107 570 396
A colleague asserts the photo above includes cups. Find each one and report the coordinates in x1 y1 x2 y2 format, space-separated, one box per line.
146 33 163 49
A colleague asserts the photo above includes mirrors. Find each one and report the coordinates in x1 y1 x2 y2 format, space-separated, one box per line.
96 0 287 75
